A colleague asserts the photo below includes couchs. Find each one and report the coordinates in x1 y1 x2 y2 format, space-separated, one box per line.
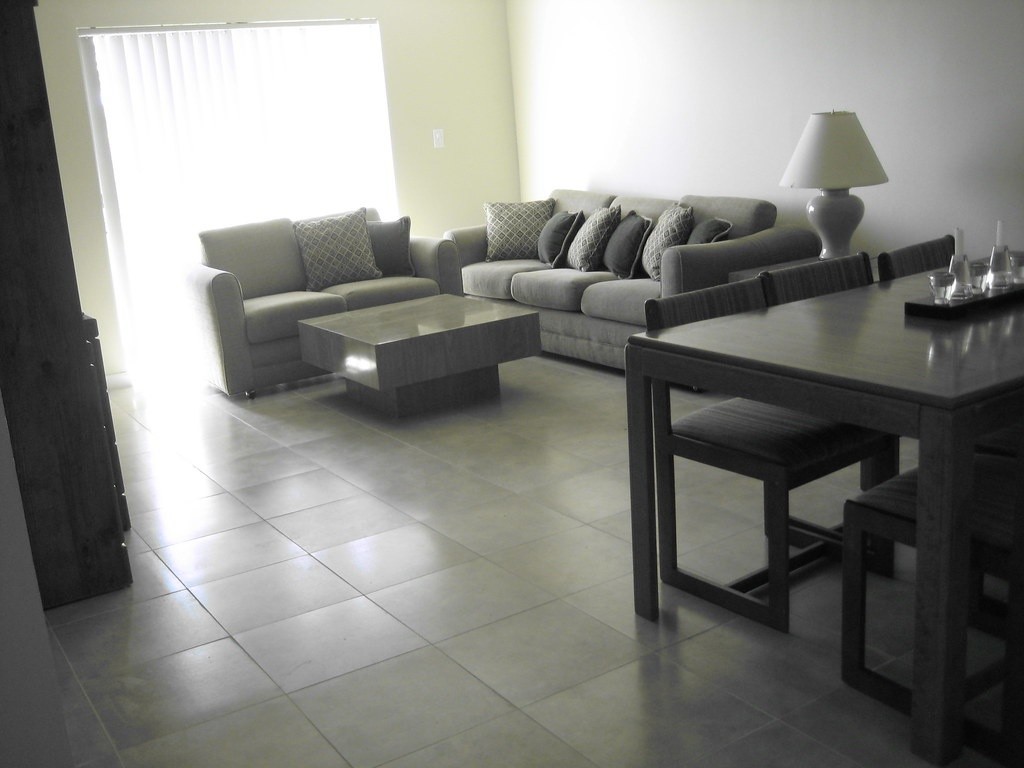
188 208 464 400
444 189 822 395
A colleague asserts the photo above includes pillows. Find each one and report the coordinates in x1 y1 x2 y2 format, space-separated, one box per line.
483 198 555 262
566 205 622 271
641 202 694 282
293 207 382 291
538 211 586 268
366 216 416 278
687 217 733 246
602 210 653 280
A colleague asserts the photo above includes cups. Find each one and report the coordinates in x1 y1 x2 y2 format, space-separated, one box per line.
969 264 989 294
1010 256 1024 283
928 272 956 304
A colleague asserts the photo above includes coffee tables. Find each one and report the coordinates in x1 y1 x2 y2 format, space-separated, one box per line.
298 294 541 419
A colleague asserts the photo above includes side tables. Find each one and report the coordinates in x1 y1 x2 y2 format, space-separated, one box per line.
727 258 880 283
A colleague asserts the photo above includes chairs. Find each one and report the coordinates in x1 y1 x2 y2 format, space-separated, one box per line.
878 234 957 282
967 422 1024 639
758 253 903 570
644 272 893 634
840 441 1024 768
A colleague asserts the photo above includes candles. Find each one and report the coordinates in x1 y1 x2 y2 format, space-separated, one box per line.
995 219 1005 254
955 227 964 262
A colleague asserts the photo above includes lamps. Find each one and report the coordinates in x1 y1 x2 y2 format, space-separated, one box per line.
779 109 889 261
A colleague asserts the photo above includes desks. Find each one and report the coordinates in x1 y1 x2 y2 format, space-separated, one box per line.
624 260 1024 765
